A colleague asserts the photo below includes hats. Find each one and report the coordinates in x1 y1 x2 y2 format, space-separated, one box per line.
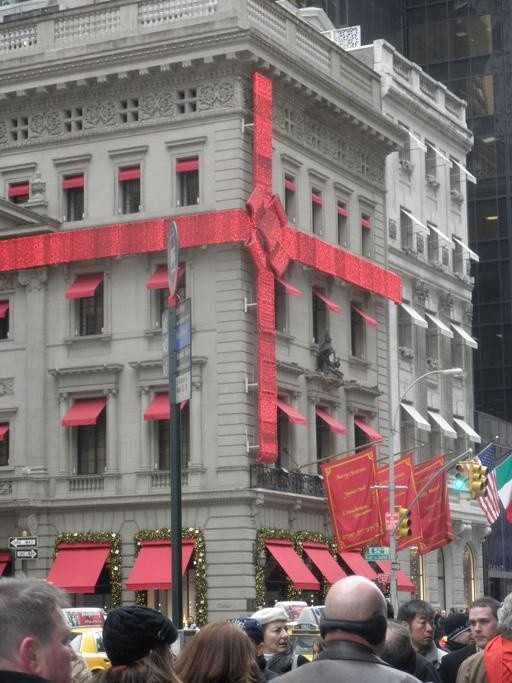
103 606 178 667
251 607 290 626
223 617 264 646
444 612 471 640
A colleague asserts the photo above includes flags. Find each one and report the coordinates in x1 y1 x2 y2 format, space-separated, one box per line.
372 450 425 549
459 438 500 526
407 454 453 556
318 442 384 553
488 448 512 522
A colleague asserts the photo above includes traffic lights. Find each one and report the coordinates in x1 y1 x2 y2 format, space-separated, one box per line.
454 460 466 490
471 466 490 499
468 461 483 492
398 505 412 539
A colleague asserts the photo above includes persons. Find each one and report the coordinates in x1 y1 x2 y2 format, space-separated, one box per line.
91 573 512 682
0 573 81 681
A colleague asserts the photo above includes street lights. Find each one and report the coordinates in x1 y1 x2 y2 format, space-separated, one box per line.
387 366 467 614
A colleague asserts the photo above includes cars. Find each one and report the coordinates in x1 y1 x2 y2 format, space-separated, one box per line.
273 597 309 636
59 605 113 674
288 605 325 662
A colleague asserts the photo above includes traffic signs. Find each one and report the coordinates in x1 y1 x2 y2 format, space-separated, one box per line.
390 562 402 571
14 548 40 560
6 535 38 549
362 547 390 561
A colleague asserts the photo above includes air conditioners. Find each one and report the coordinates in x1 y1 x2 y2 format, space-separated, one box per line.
402 159 410 169
426 175 435 183
